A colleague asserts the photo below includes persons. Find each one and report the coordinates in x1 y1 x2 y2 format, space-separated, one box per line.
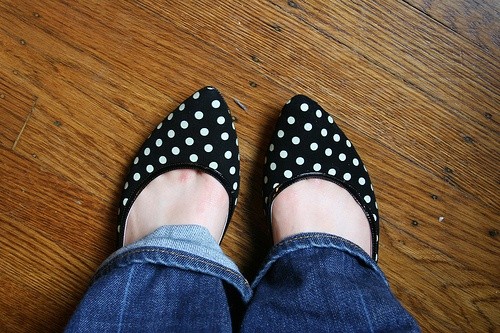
62 86 423 333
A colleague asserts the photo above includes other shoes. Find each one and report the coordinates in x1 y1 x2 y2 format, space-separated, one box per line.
116 86 241 249
262 94 379 265
